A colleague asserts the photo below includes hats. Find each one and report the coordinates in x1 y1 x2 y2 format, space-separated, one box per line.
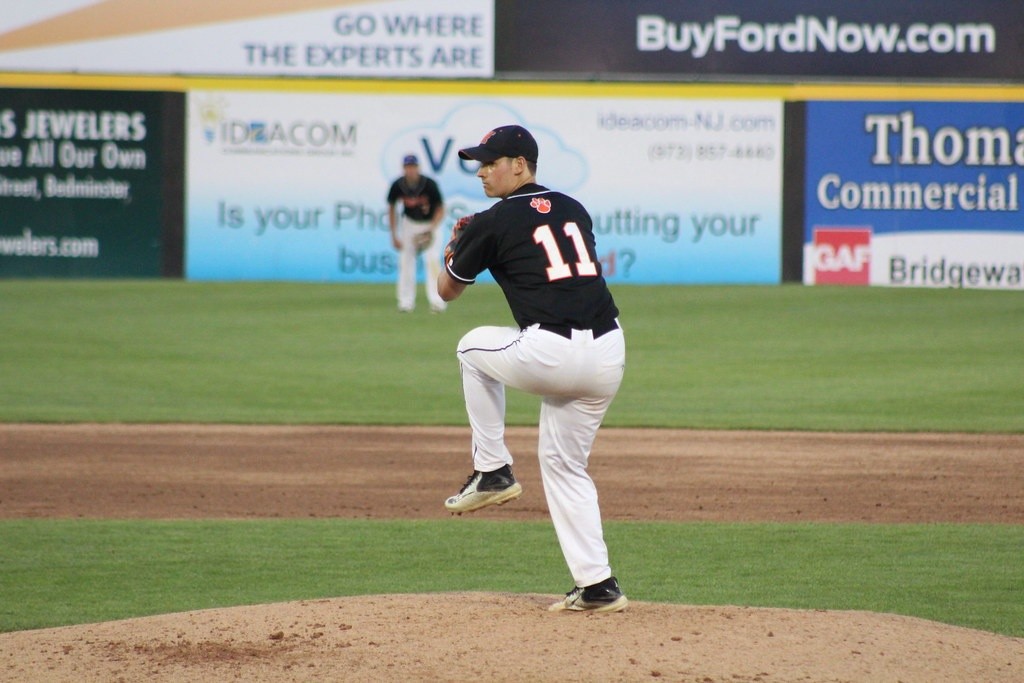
458 125 538 164
404 156 418 165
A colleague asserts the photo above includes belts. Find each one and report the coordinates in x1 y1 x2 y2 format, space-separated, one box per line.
539 318 619 340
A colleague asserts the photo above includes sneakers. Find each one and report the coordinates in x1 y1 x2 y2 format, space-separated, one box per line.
548 577 628 612
445 464 523 516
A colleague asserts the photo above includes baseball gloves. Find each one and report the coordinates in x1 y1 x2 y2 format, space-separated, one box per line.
441 213 478 267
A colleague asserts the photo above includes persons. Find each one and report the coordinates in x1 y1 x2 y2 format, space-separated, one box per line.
438 124 629 612
387 154 448 314
